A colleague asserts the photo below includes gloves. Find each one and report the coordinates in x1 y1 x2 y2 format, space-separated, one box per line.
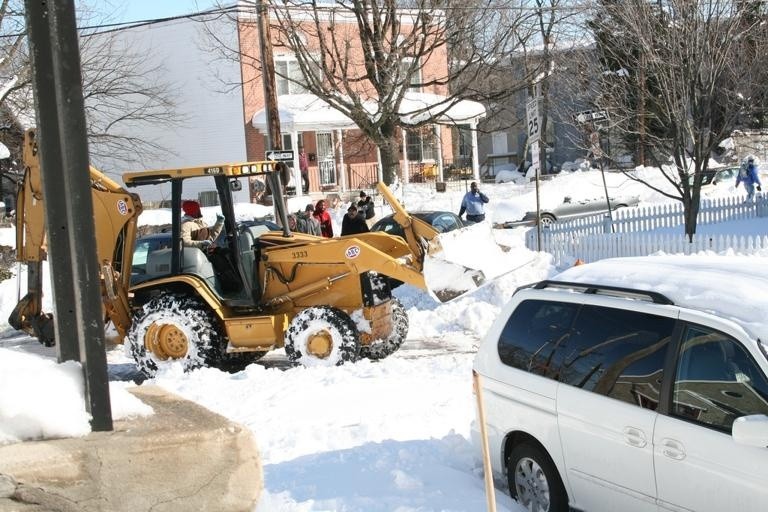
203 240 212 246
217 214 225 223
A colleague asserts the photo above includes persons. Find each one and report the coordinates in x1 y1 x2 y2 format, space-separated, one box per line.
181 200 241 293
299 145 309 194
735 155 761 204
297 191 377 238
459 181 489 223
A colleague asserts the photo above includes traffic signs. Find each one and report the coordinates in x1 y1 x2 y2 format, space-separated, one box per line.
570 107 609 126
265 150 294 161
526 98 540 144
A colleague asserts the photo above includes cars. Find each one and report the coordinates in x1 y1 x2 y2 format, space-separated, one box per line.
368 211 476 289
678 166 741 189
491 172 640 229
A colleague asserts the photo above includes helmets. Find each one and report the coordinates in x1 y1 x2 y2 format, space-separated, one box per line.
183 200 202 218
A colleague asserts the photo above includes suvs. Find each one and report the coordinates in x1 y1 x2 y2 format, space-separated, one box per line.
471 256 767 512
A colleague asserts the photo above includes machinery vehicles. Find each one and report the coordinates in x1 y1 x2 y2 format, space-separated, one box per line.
7 126 536 378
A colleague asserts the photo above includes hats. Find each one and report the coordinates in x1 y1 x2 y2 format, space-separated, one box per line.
306 204 314 211
360 191 366 197
348 202 357 211
